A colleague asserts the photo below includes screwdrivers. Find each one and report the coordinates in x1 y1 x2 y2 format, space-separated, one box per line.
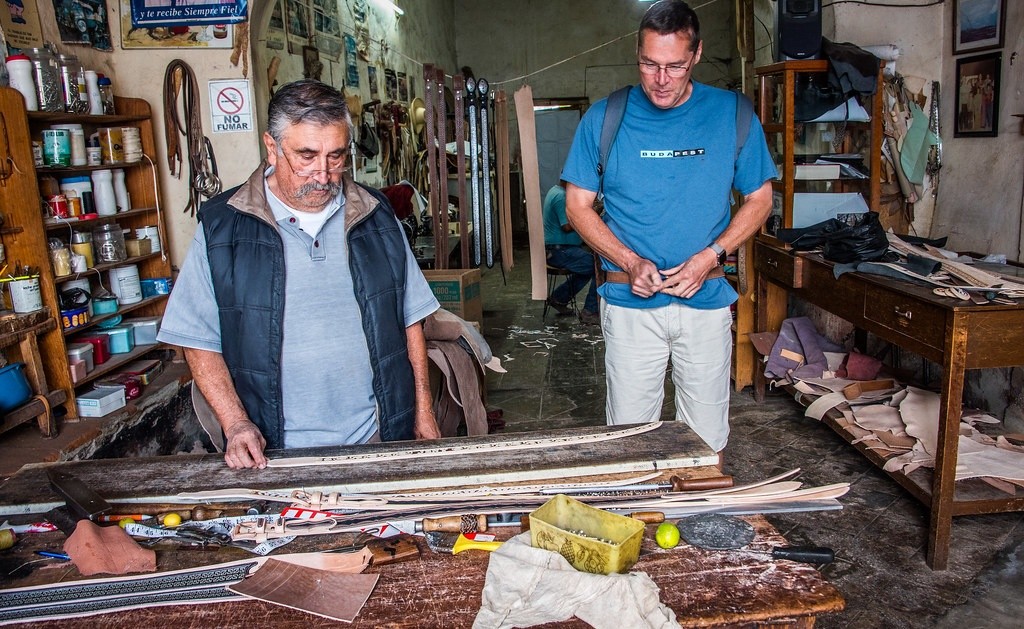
712 546 835 563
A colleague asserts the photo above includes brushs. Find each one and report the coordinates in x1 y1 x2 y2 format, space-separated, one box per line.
426 532 505 555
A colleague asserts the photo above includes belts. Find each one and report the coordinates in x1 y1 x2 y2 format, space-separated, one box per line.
606 267 725 284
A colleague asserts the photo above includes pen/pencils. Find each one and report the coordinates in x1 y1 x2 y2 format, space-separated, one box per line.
98 515 153 521
33 551 71 559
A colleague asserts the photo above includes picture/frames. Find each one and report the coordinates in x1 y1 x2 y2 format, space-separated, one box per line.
952 0 1007 137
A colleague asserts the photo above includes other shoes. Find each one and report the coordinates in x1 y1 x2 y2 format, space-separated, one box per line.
547 297 574 315
580 310 599 324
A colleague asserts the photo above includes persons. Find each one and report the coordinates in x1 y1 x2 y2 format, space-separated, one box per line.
561 0 779 472
155 78 442 469
543 168 600 324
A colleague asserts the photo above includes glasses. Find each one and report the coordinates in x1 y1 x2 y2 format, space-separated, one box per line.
274 137 352 177
636 42 695 78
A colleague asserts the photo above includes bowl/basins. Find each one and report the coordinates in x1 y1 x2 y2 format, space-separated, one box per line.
93 296 120 314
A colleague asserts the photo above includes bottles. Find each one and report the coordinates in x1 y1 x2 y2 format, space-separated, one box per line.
5 47 115 115
31 124 143 167
49 224 160 276
37 169 131 219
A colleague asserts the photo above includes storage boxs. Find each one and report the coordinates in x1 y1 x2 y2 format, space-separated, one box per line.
76 237 174 418
417 270 483 330
531 494 647 574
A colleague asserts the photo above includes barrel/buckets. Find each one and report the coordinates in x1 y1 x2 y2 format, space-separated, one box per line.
7 277 43 313
109 264 142 304
59 277 94 317
0 362 33 411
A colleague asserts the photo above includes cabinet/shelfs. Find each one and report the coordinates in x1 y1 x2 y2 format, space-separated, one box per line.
0 88 185 422
756 61 882 248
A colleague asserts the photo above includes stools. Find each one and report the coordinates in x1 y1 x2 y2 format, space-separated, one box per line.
543 265 583 323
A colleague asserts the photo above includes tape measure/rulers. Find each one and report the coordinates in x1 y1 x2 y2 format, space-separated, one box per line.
125 508 363 556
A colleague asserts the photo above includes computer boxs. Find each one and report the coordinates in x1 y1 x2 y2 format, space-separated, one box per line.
771 0 823 63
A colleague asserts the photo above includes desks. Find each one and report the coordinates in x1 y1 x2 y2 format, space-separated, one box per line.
754 240 1024 571
1 421 845 629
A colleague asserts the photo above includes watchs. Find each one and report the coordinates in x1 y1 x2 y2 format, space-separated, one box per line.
708 241 727 266
